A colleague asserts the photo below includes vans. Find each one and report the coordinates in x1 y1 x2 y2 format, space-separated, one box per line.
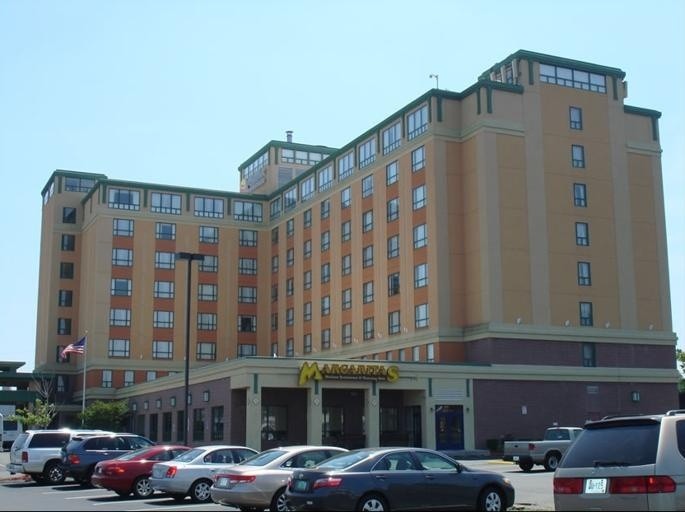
7 428 114 484
553 410 684 511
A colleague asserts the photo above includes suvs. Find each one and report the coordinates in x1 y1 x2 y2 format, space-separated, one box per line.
61 433 156 488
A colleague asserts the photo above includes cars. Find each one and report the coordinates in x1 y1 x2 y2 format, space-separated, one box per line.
284 446 514 511
149 445 260 503
90 445 193 498
210 445 351 512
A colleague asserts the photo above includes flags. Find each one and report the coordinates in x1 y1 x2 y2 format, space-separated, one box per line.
61 337 85 359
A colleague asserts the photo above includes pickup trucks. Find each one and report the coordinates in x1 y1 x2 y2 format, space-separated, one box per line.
502 427 583 471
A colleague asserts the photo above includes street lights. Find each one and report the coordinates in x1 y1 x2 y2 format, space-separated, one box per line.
175 251 204 443
